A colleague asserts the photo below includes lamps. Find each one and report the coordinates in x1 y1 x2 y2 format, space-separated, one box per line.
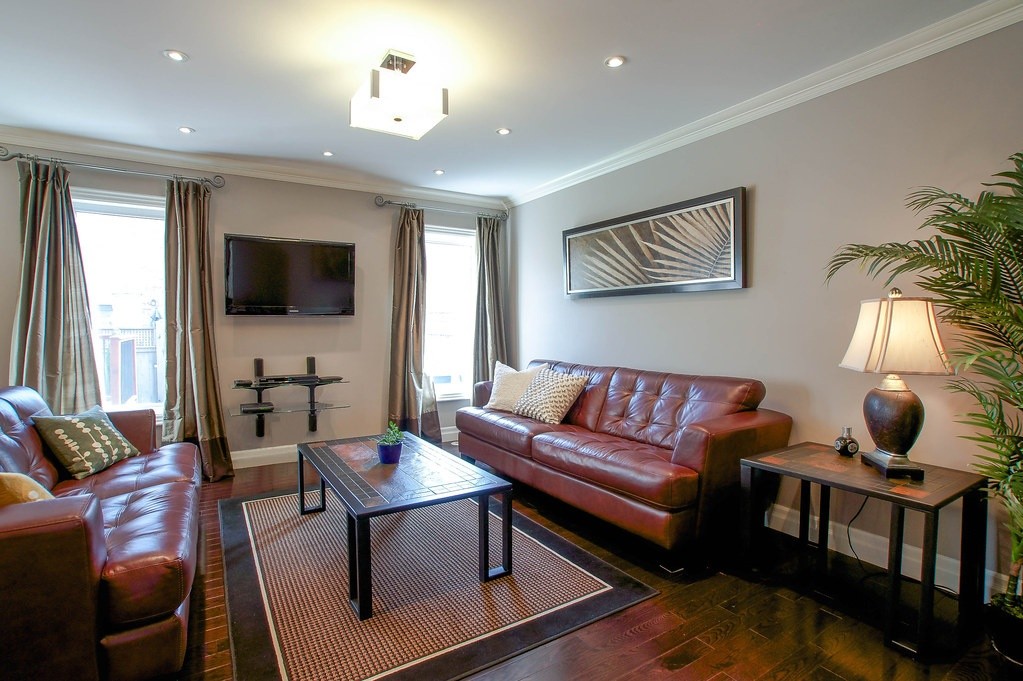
345 51 452 144
839 286 959 489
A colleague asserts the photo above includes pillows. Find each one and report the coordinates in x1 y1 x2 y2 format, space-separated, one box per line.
32 402 142 481
482 361 588 426
0 473 56 509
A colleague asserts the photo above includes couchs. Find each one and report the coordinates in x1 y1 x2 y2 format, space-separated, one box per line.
455 359 793 576
0 382 203 681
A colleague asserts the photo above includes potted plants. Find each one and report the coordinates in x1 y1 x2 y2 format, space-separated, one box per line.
374 424 404 466
823 147 1023 681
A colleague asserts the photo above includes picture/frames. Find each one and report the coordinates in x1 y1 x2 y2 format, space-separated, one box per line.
560 184 749 301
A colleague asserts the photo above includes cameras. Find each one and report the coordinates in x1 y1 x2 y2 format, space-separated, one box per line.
223 232 356 317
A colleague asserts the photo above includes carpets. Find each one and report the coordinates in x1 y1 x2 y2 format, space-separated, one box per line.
215 469 660 679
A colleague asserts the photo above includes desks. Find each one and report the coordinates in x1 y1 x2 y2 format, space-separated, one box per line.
294 426 515 622
735 439 993 662
